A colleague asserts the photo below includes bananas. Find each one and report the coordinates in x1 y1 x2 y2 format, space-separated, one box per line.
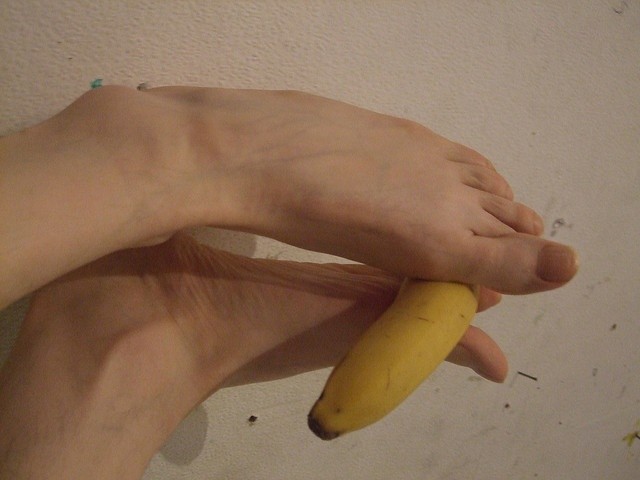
307 269 484 440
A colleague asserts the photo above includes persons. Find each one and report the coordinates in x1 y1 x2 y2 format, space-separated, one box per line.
1 79 579 480
